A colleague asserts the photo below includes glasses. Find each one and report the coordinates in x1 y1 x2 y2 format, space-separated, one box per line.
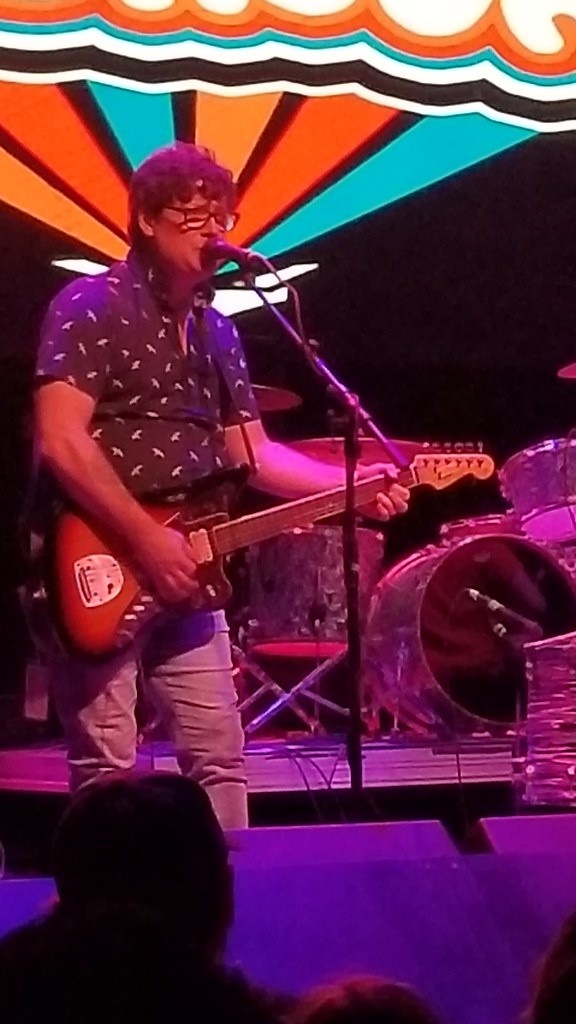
160 199 239 232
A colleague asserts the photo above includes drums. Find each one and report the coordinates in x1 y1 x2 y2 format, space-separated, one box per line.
495 436 575 543
360 531 576 741
238 521 387 660
438 513 514 540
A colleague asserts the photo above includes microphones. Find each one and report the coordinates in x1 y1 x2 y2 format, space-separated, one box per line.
204 236 268 273
468 588 543 640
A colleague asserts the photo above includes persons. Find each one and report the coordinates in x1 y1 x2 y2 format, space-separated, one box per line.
0 774 574 1023
34 141 411 829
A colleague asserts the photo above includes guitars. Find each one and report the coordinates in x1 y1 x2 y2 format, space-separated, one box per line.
42 441 497 661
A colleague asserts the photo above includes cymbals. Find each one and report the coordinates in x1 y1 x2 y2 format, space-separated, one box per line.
249 383 304 412
284 435 427 473
557 360 576 379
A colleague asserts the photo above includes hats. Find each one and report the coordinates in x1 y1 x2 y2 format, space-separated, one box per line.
53 770 238 905
